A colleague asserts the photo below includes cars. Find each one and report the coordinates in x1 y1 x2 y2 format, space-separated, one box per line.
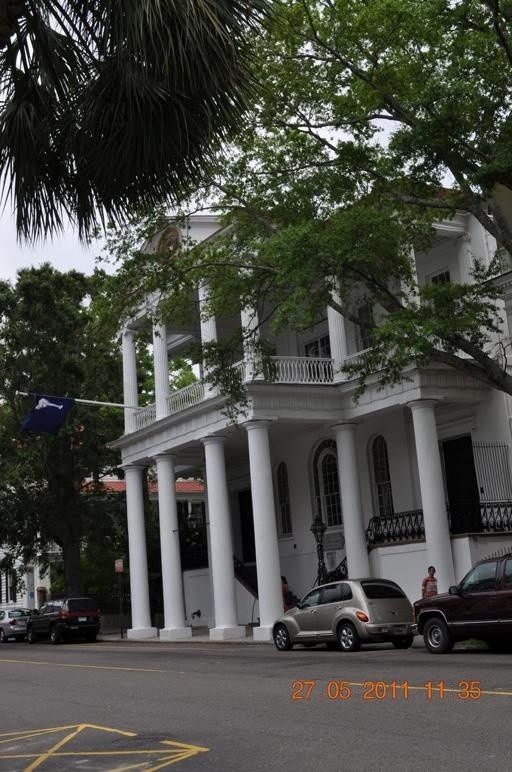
414 554 512 653
0 608 34 643
273 578 419 652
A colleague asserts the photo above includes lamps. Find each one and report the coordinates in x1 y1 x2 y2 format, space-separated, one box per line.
185 511 209 532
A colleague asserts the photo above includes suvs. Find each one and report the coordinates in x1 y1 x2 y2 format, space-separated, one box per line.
26 598 101 644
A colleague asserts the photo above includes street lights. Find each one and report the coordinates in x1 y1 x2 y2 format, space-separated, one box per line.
310 515 329 587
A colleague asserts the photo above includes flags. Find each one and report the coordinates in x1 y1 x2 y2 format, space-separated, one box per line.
19 394 74 436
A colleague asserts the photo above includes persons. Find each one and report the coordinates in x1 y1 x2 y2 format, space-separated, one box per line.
421 566 438 599
